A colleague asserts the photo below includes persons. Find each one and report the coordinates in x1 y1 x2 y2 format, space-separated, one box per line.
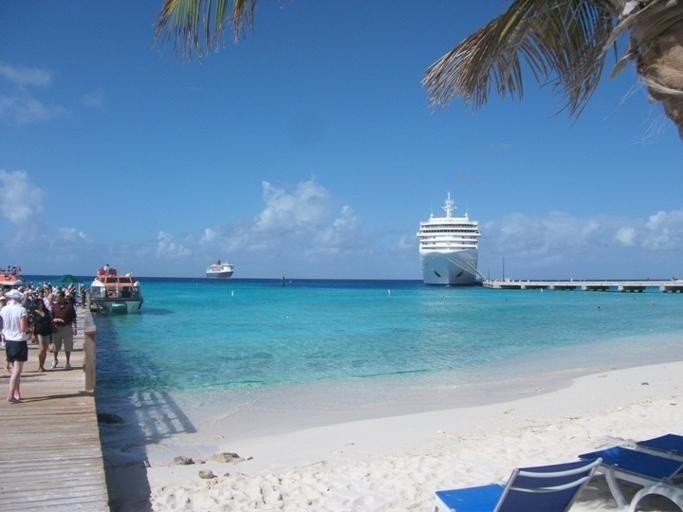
0 261 140 405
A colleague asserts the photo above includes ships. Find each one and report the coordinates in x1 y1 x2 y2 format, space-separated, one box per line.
415 189 481 287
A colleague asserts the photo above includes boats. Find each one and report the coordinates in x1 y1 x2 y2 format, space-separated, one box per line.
205 261 235 280
89 268 144 315
0 264 27 289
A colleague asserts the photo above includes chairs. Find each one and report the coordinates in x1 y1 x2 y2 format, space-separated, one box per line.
579 428 682 512
430 454 603 512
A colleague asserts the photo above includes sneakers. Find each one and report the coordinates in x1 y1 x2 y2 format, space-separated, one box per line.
65 363 72 370
51 359 58 368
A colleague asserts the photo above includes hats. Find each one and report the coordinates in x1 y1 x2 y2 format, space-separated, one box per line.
4 289 24 299
